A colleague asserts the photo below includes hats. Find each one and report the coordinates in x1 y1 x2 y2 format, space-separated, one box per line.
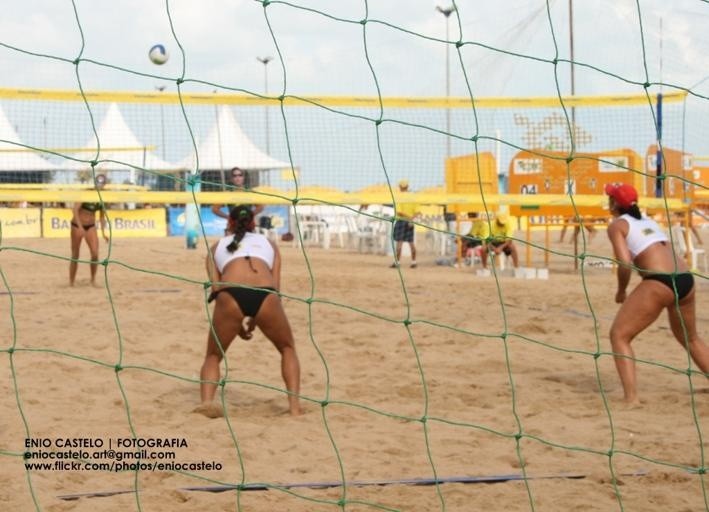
605 182 638 207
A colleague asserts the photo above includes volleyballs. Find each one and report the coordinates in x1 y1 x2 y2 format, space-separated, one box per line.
149 44 170 63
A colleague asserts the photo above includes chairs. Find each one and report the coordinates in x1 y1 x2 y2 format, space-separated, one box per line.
671 227 708 273
290 204 512 272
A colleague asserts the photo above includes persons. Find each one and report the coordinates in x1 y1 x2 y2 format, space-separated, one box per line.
195 204 306 418
481 213 519 267
68 175 108 288
209 166 264 235
387 179 422 268
452 213 489 268
604 183 708 404
356 205 374 248
